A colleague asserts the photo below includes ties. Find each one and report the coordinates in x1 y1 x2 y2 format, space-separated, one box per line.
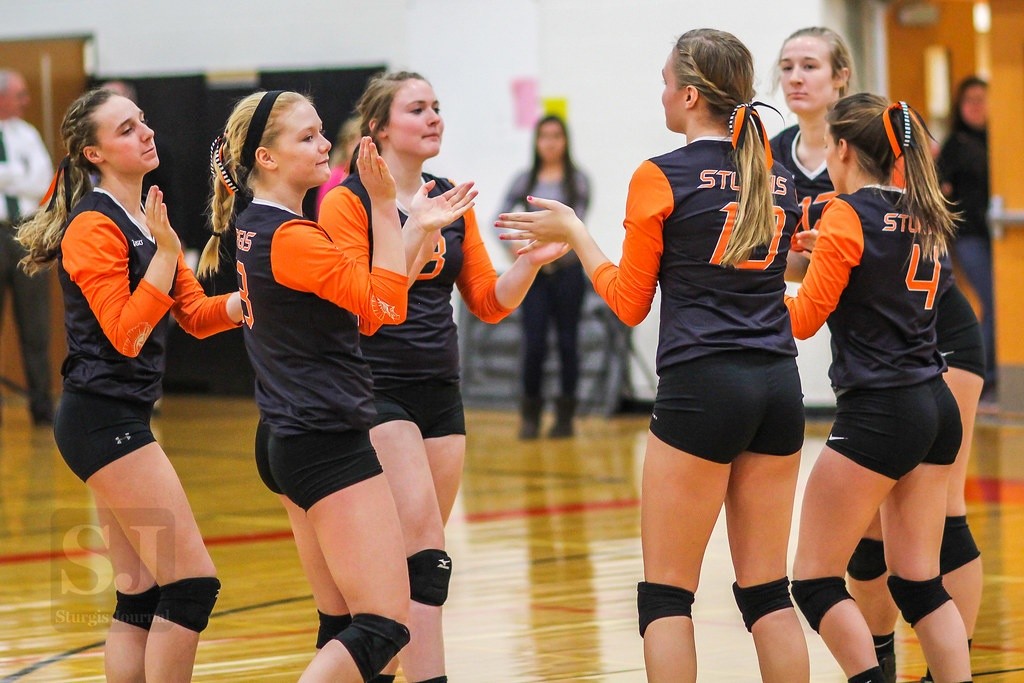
0 133 20 226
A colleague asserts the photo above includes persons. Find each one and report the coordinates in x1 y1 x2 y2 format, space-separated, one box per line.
489 108 594 438
19 27 984 683
1 64 56 427
929 75 996 405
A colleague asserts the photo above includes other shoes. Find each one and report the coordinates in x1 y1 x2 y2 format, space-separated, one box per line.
30 412 53 426
549 423 576 439
518 421 538 441
